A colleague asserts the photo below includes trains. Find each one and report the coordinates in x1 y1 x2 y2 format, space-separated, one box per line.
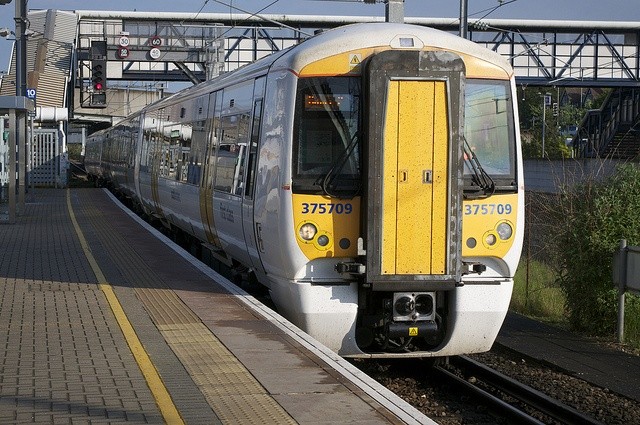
84 22 525 359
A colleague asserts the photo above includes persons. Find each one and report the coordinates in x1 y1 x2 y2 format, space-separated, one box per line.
186 155 199 185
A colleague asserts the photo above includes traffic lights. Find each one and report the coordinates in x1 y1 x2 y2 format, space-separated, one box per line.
92 60 106 92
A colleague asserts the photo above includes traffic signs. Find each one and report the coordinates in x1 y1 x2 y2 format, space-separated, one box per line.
150 36 161 47
120 36 130 46
